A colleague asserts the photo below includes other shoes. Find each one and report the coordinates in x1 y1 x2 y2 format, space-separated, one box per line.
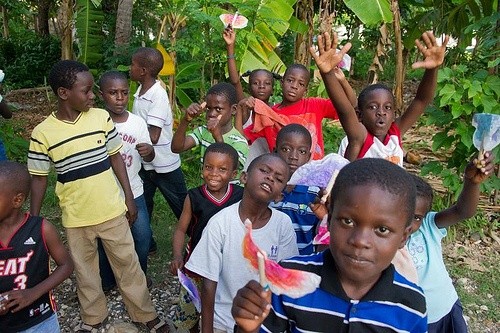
148 236 157 253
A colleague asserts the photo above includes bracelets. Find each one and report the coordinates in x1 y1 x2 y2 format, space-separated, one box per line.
226 54 236 60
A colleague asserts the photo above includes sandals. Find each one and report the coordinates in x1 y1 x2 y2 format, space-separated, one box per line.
142 316 165 332
80 316 108 332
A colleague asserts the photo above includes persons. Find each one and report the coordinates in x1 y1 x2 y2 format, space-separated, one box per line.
184 153 299 333
230 157 428 333
267 123 325 253
223 23 277 172
309 187 419 285
402 152 496 333
0 161 74 333
128 47 192 239
27 58 170 333
309 30 450 166
233 65 358 161
169 81 247 187
95 71 154 292
